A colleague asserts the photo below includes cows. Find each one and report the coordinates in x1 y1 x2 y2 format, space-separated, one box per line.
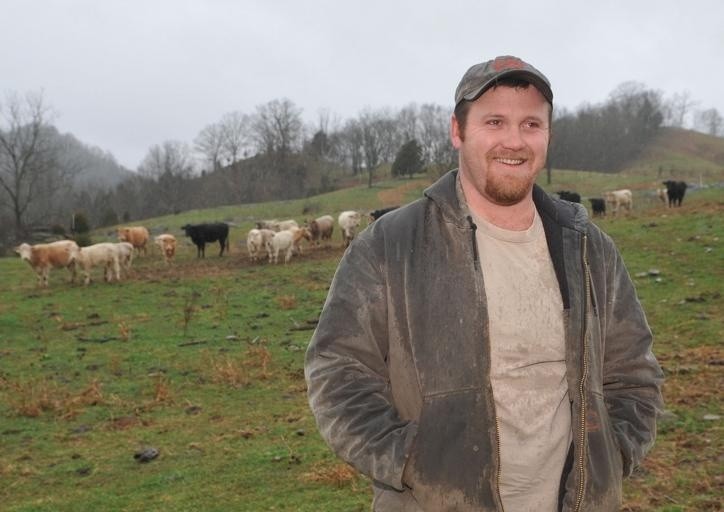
15 239 134 289
588 198 606 217
656 181 688 208
370 206 399 220
181 223 230 259
337 210 365 249
119 226 150 259
154 233 177 268
556 191 581 205
246 215 335 265
604 189 632 217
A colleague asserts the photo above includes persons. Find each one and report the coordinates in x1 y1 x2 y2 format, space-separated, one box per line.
303 54 663 511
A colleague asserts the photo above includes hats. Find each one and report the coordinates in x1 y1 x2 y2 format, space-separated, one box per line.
452 55 554 107
